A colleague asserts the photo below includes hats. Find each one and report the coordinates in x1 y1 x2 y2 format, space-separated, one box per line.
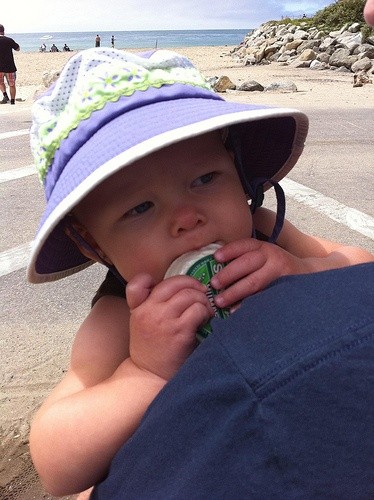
28 45 309 283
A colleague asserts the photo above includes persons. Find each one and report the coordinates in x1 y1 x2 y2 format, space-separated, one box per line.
27 47 374 497
40 44 46 51
51 44 58 52
77 0 374 500
0 24 20 104
64 44 70 51
111 35 115 48
96 35 100 47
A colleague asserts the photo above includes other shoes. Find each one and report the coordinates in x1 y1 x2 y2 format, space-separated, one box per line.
0 95 9 104
